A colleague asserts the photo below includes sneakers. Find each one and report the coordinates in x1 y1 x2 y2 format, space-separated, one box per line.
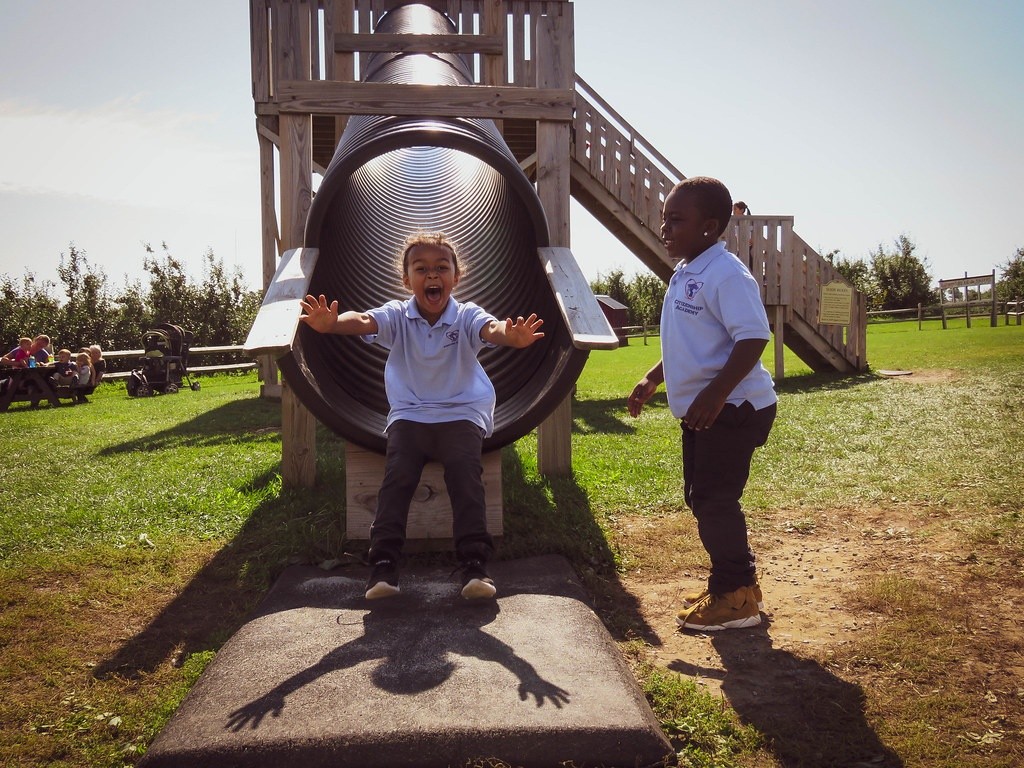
673 586 762 632
460 571 496 599
365 560 401 600
681 584 764 612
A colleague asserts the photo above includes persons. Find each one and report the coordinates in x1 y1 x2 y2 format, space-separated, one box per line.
297 231 545 601
732 198 754 273
623 174 779 633
0 333 107 411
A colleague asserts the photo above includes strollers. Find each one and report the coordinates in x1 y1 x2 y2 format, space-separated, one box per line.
126 323 200 397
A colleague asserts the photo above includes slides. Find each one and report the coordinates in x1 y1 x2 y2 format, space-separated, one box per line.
240 1 622 461
